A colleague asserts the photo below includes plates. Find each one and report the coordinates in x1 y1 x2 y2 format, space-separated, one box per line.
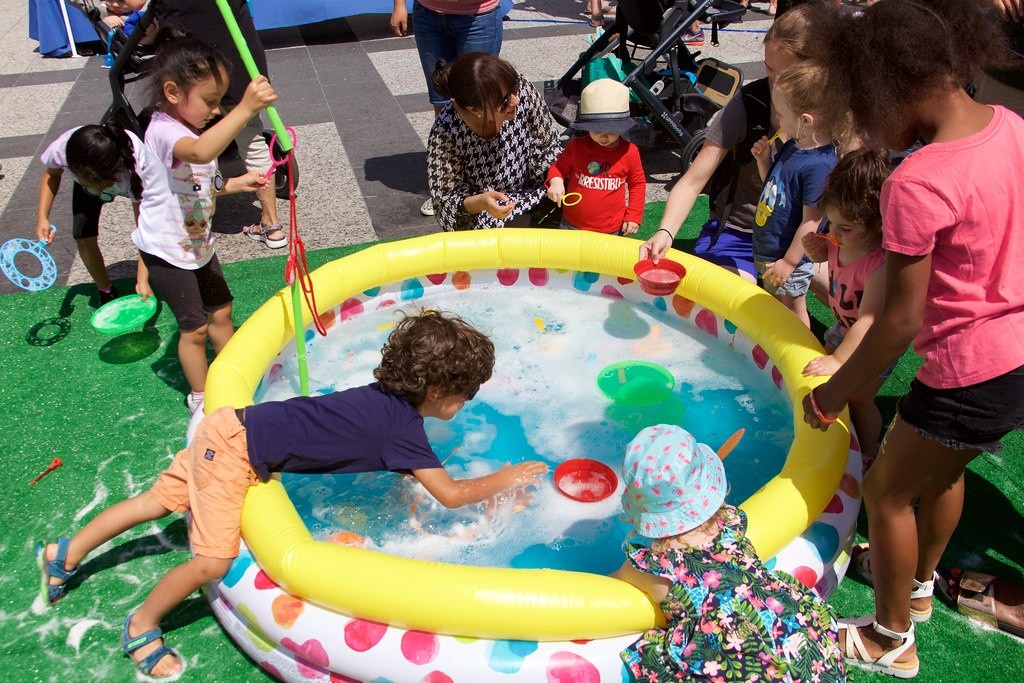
90 293 157 334
598 361 676 407
554 458 618 503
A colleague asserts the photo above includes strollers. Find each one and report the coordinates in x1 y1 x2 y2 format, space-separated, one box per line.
541 0 746 175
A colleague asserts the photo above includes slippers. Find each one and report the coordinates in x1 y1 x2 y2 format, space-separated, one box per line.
936 567 1024 640
579 8 608 17
589 14 606 28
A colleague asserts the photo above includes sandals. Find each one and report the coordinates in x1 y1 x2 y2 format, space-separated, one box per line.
36 538 81 607
120 613 184 679
837 612 920 678
853 542 940 622
243 221 288 249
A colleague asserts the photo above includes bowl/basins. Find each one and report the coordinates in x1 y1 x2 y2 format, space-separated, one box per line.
633 259 686 296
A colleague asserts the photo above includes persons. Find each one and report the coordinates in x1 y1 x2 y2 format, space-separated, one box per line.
544 78 646 237
639 0 1024 679
131 27 277 415
98 0 288 249
584 0 616 27
390 0 503 216
36 124 152 307
426 52 565 232
619 423 853 683
37 312 549 680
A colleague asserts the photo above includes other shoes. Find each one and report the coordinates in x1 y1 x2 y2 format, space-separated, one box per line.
98 283 119 304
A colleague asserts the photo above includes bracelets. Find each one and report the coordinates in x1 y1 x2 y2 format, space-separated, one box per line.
655 229 674 244
809 388 838 424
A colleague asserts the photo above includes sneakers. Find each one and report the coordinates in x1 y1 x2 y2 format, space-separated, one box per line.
421 197 435 216
680 28 704 46
187 389 205 417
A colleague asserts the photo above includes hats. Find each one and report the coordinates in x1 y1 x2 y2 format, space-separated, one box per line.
569 78 638 134
621 424 727 539
